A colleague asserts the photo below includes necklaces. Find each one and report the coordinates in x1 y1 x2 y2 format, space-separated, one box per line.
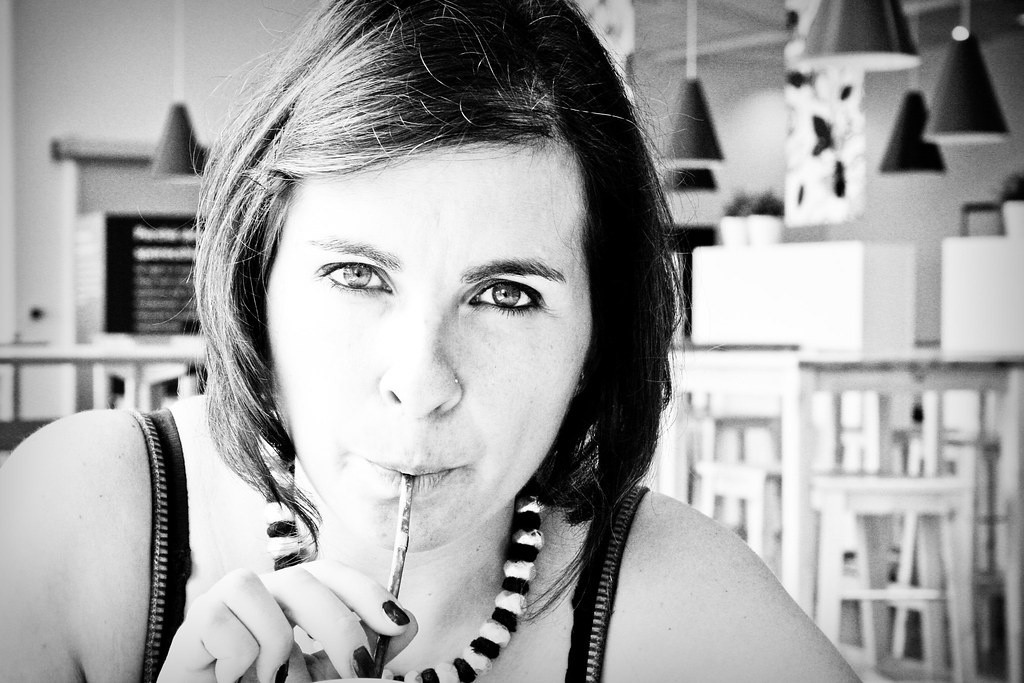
259 454 546 683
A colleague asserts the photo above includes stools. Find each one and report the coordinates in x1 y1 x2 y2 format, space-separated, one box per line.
692 458 785 576
817 474 979 683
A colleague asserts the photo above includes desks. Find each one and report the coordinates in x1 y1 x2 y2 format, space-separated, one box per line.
796 355 1024 683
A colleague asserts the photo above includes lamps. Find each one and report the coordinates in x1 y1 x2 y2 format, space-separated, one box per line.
655 1 729 251
924 0 1010 142
151 0 206 180
797 0 922 75
877 16 951 177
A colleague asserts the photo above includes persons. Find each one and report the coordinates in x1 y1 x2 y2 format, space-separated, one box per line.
0 0 863 683
